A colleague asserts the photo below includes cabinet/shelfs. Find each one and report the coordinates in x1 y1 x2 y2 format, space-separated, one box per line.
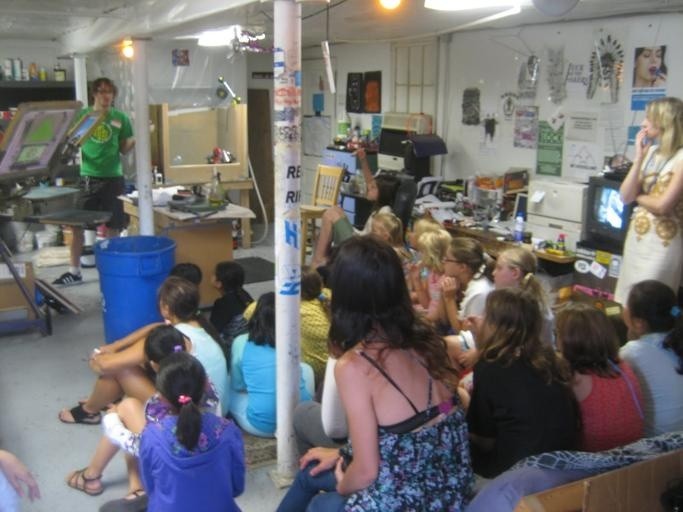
322 145 377 231
571 251 619 292
408 214 574 277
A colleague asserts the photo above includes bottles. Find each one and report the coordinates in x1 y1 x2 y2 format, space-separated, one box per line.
514 211 524 241
1 57 66 83
231 220 238 249
556 234 565 251
207 176 225 208
8 107 16 120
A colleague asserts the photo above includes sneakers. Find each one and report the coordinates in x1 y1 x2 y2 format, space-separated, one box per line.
51 271 82 288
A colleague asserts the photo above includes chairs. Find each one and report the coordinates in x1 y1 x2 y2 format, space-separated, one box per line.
299 165 345 261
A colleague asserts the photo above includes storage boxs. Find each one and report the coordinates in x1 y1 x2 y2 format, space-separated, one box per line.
0 260 34 324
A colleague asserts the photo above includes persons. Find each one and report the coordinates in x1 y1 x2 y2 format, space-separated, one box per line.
58 262 334 512
49 78 156 290
314 205 581 491
349 147 378 202
614 97 683 308
558 279 683 449
277 233 477 512
0 448 41 512
633 45 667 90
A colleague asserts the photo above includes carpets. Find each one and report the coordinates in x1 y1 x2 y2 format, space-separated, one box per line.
228 423 283 472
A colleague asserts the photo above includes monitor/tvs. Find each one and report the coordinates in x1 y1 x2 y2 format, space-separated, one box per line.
586 177 633 246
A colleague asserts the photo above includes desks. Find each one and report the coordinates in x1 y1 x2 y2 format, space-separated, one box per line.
214 179 254 247
0 208 113 337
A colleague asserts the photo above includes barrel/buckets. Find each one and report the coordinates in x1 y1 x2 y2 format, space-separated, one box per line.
92 235 177 352
15 224 96 252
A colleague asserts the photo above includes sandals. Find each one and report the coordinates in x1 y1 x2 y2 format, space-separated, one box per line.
59 404 101 425
125 489 146 501
65 468 103 495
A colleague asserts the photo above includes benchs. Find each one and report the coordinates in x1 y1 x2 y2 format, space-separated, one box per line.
463 432 682 512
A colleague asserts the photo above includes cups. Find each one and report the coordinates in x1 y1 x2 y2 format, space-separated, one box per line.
54 176 64 188
0 111 9 120
342 183 352 195
337 120 351 135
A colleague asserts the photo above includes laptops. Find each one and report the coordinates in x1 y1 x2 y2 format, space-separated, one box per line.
499 194 529 231
